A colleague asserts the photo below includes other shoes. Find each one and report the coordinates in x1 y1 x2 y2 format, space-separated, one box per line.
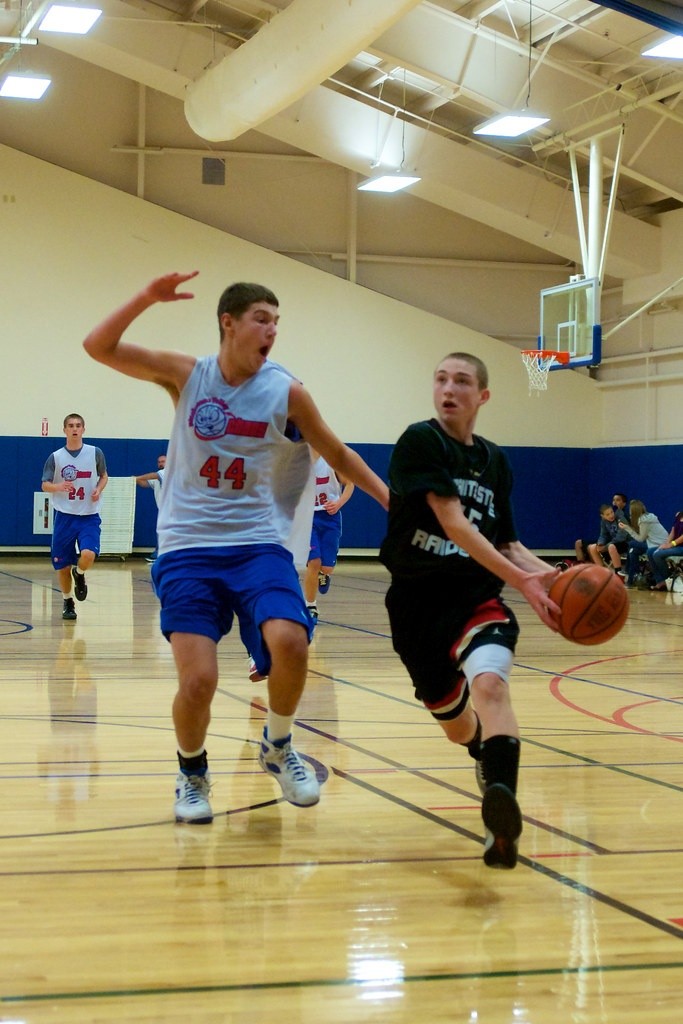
649 584 668 591
146 556 158 562
618 570 630 576
624 584 633 589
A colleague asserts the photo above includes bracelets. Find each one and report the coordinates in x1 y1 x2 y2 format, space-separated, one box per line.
671 540 676 546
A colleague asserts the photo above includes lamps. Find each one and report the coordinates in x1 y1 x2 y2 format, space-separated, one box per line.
38 2 104 37
473 0 552 140
640 32 683 59
0 1 51 100
356 70 422 193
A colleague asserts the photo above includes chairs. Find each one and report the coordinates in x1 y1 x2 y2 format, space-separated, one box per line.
602 551 683 592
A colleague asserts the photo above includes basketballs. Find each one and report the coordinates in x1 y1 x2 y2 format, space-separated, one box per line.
547 564 632 646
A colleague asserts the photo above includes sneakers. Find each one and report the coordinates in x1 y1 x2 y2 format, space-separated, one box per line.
318 570 330 594
250 656 267 681
62 598 77 620
475 758 488 796
306 604 319 624
72 566 87 601
259 727 321 808
174 772 213 824
481 784 522 869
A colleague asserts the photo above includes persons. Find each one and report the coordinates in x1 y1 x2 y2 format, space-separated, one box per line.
84 266 388 825
383 352 566 871
136 447 353 682
41 414 109 618
575 493 683 590
